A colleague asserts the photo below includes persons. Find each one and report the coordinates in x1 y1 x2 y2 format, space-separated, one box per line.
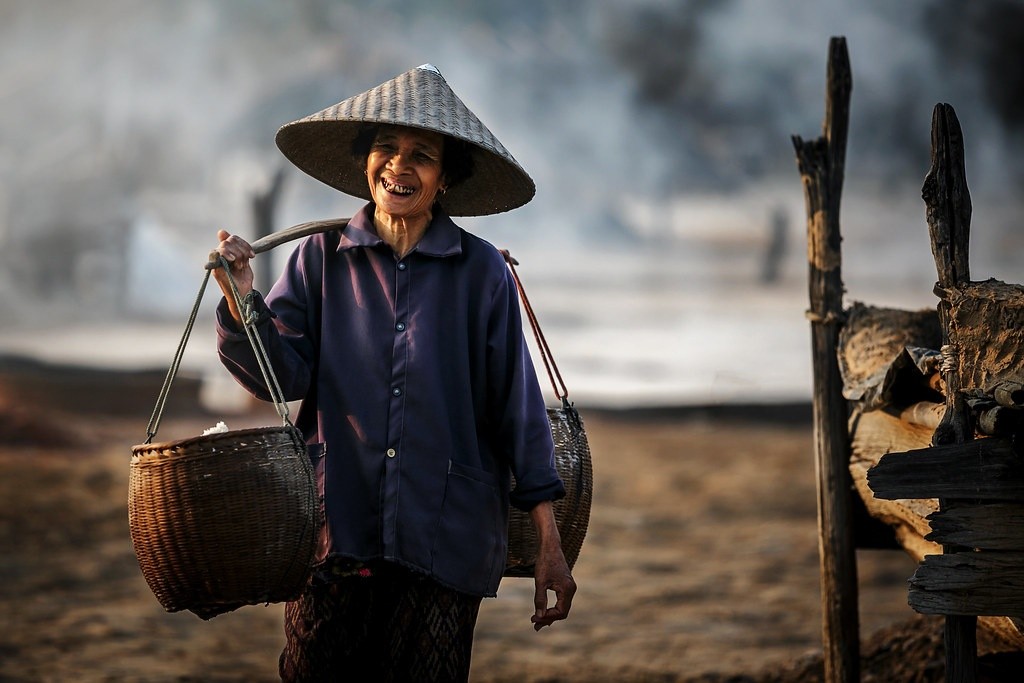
211 62 577 683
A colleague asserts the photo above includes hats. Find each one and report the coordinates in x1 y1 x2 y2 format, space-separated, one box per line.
274 62 536 217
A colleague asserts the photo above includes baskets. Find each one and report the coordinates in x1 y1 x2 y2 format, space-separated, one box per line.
500 405 594 579
127 425 321 622
